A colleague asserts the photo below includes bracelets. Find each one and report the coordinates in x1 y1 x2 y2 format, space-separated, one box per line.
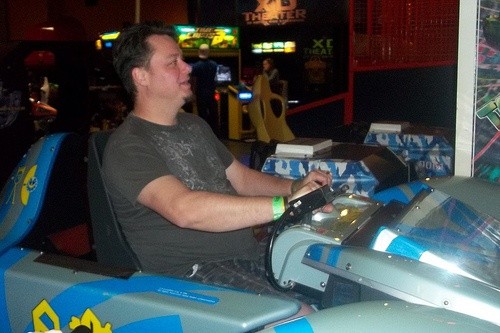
291 180 300 194
272 194 286 222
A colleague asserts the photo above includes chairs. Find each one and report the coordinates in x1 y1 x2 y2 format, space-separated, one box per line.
248 74 296 145
85 130 144 272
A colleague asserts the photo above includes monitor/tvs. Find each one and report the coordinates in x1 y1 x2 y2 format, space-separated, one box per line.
214 65 231 81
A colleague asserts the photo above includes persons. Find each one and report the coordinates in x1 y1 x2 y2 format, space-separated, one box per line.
190 41 216 134
260 57 281 99
103 21 336 329
